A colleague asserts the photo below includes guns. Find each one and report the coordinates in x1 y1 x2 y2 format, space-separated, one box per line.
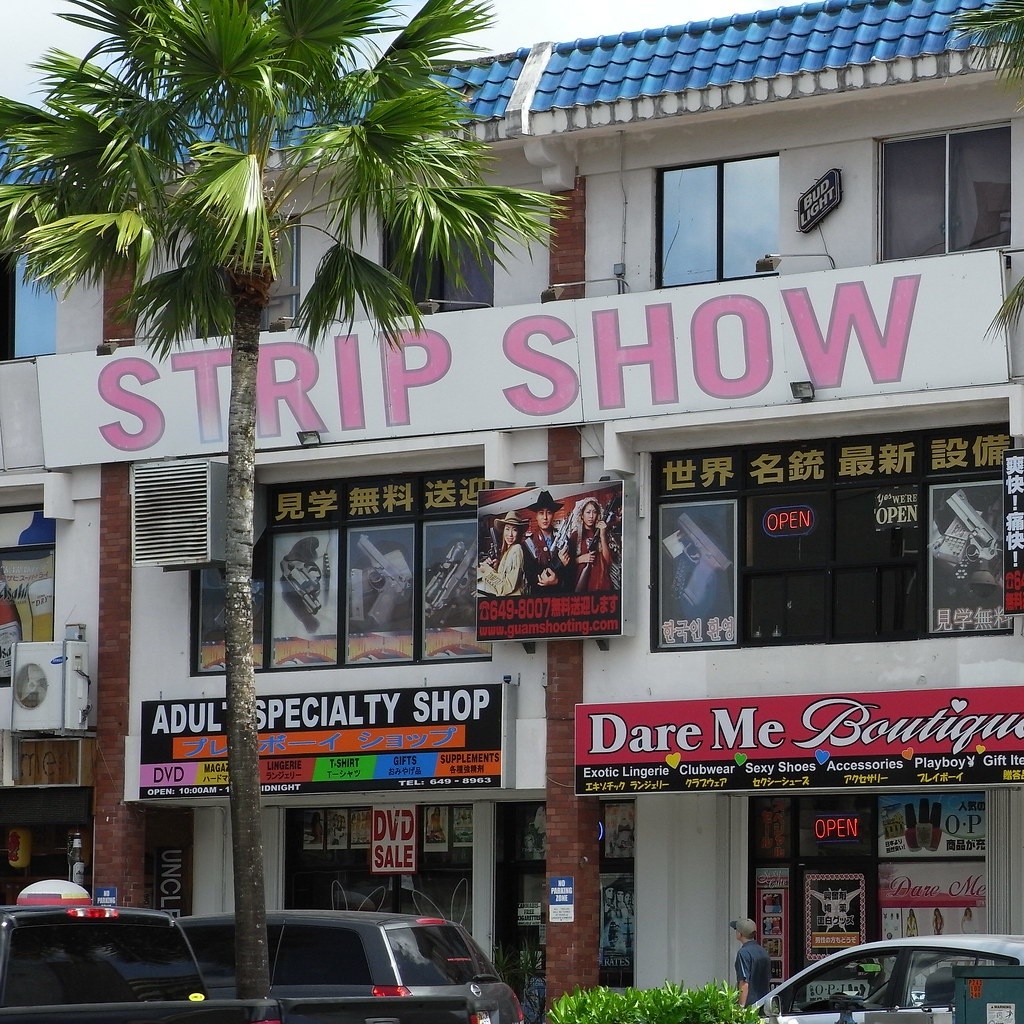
678 512 732 607
280 536 325 615
356 535 410 628
425 539 476 629
480 527 500 562
947 490 1003 563
551 512 575 555
575 486 621 595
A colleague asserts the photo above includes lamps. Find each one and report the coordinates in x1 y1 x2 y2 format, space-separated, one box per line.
268 319 293 333
790 380 815 403
541 287 565 304
415 302 439 315
97 342 120 356
754 254 782 273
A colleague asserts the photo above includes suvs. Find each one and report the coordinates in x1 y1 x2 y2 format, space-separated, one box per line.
175 910 527 1024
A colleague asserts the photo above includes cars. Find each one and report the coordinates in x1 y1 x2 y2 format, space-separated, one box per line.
744 931 1024 1024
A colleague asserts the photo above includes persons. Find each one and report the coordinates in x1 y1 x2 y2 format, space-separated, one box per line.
569 497 614 596
331 814 347 837
521 805 548 859
731 918 773 1008
526 491 577 597
609 817 633 858
310 812 323 847
602 884 635 922
479 512 524 599
430 807 445 842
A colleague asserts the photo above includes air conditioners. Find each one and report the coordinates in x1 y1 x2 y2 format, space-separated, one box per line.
10 640 89 734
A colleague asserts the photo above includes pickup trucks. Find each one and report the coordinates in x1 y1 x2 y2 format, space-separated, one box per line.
1 903 484 1024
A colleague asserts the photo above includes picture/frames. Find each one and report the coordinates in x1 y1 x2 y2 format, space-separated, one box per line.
509 801 546 864
748 862 794 1015
451 804 473 848
927 479 1014 634
876 791 987 860
197 528 267 672
878 861 988 941
658 499 737 648
600 799 634 865
325 807 350 850
795 862 876 1005
599 865 634 973
345 523 415 665
749 796 796 861
302 808 325 851
268 528 338 668
422 518 493 660
422 805 450 854
350 807 373 850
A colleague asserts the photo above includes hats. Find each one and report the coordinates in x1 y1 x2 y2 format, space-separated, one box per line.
728 919 757 937
493 511 530 535
526 490 564 513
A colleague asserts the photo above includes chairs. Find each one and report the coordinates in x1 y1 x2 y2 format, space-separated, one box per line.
920 972 955 1006
